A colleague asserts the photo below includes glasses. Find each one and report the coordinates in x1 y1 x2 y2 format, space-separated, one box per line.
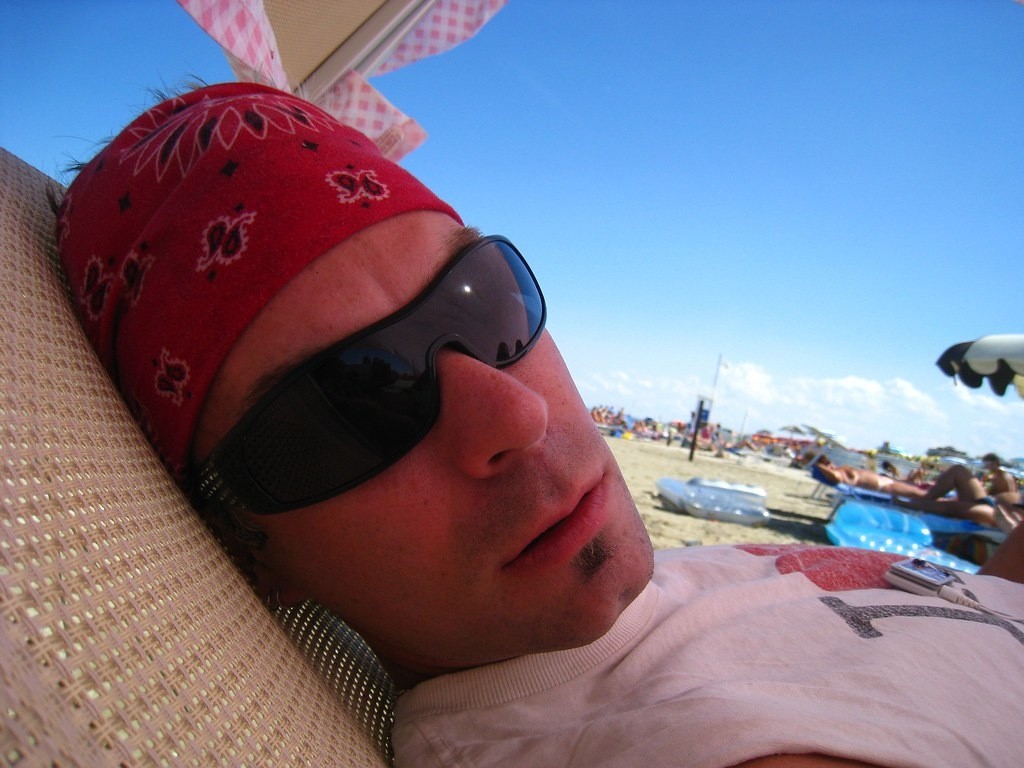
188 235 548 514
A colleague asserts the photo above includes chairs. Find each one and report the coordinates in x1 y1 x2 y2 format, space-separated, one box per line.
1 146 402 767
809 463 910 500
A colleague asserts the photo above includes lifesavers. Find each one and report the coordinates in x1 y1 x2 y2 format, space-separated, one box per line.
680 484 769 525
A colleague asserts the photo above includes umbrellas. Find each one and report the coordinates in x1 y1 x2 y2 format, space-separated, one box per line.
937 335 1024 406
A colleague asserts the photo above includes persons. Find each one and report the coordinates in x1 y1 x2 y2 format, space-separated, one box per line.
983 453 1018 496
591 405 935 488
817 455 929 500
60 81 1024 768
893 465 1023 533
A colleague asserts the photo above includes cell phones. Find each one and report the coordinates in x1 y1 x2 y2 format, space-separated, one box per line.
884 557 955 597
